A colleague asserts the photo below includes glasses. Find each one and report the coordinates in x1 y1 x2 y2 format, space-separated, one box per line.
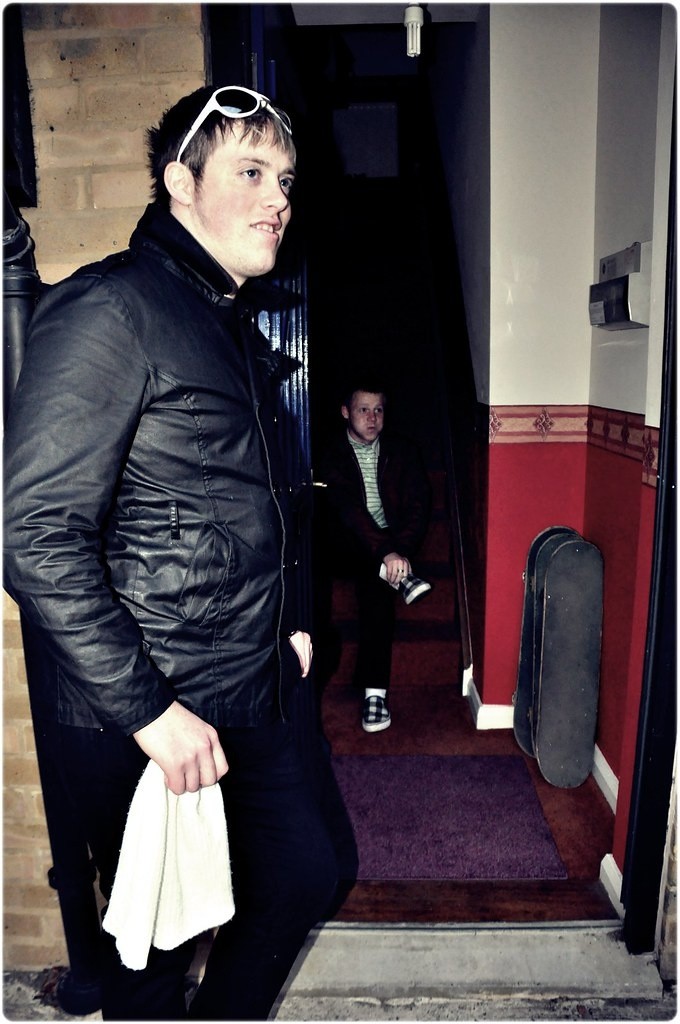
175 84 293 160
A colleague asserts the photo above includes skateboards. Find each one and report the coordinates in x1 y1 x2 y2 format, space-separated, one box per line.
512 526 605 790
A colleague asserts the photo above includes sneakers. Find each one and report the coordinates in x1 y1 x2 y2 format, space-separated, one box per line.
394 573 431 604
361 694 391 732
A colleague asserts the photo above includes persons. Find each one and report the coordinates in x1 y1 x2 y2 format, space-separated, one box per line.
7 82 361 1020
311 376 431 733
